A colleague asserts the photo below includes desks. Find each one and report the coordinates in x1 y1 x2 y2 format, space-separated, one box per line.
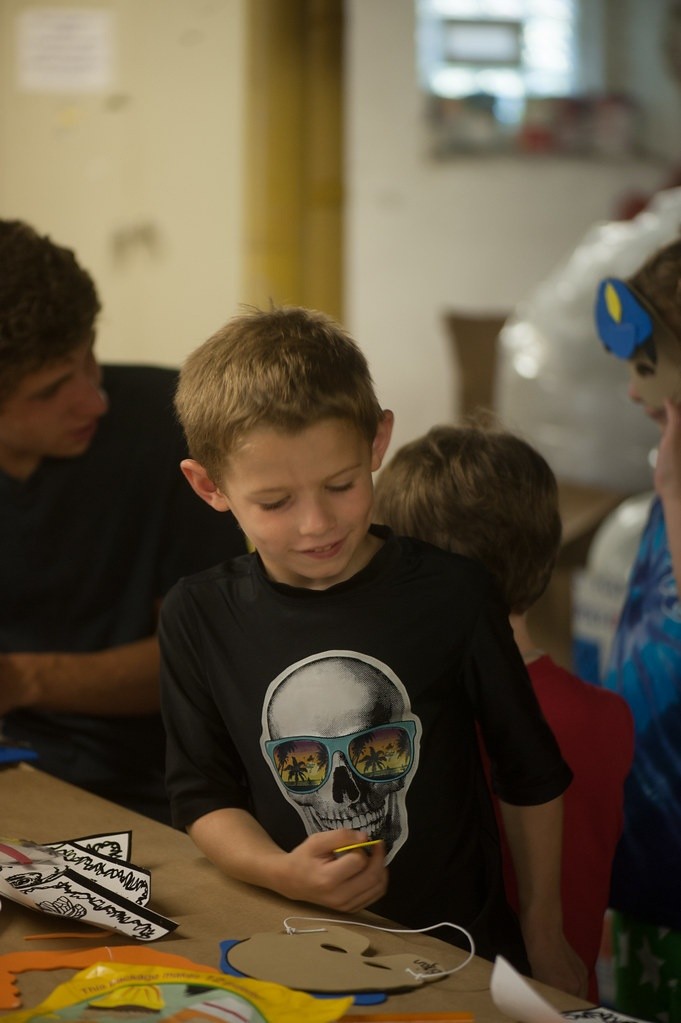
0 760 618 1023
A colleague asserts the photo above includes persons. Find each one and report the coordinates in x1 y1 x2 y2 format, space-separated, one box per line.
554 239 681 1023
0 216 238 824
368 423 638 1012
155 305 592 999
